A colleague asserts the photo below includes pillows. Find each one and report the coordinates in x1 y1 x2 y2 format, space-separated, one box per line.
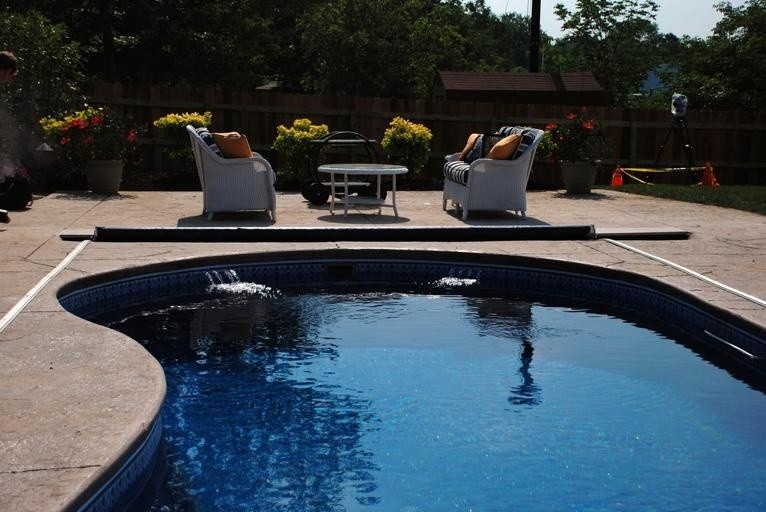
458 132 523 163
196 126 252 159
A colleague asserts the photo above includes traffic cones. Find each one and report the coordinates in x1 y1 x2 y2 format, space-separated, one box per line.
610 161 624 187
702 161 721 187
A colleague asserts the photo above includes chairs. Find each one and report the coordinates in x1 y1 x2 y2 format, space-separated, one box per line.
186 124 278 223
443 126 544 221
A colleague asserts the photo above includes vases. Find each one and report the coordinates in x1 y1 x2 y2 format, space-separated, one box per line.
560 157 602 194
64 156 85 191
84 158 124 195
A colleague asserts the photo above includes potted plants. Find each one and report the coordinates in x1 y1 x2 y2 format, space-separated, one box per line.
272 118 329 177
153 111 215 173
381 117 434 187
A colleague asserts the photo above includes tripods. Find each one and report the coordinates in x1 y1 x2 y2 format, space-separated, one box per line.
643 117 702 187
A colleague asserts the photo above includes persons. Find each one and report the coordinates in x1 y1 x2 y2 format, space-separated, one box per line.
0 51 18 84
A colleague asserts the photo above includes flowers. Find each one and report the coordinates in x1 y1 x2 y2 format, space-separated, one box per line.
541 107 611 161
39 105 101 159
60 103 141 159
